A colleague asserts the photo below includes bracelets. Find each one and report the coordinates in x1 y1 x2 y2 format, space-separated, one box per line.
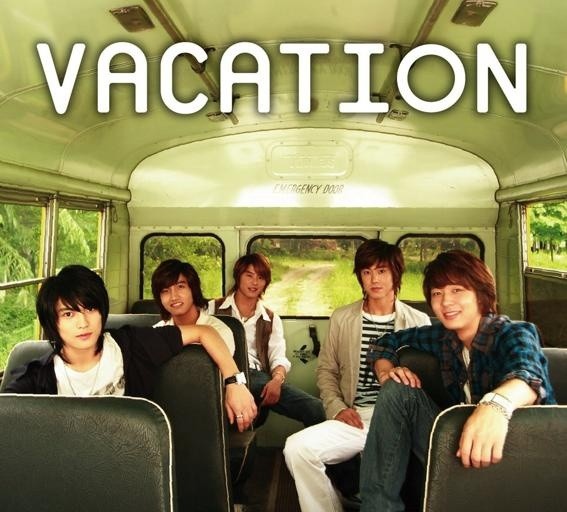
475 391 515 416
221 369 250 391
270 372 285 385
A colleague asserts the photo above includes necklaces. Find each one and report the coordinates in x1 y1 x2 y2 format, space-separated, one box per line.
366 301 394 339
234 301 254 322
63 349 101 396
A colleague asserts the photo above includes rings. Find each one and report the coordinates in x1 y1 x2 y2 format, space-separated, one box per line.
236 413 244 420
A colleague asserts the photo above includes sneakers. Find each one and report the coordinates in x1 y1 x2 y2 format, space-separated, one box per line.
341 492 360 505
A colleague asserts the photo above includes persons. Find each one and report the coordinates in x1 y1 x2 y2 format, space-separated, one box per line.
278 238 432 512
354 247 557 511
1 265 259 436
148 256 235 355
197 254 365 508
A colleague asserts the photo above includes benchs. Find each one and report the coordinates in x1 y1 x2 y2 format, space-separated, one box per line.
1 299 258 512
352 299 567 511
